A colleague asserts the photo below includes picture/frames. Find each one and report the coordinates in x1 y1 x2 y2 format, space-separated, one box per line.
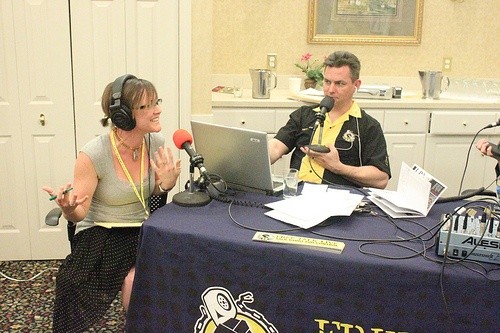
308 0 425 46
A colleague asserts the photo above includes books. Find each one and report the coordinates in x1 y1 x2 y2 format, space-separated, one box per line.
367 162 448 218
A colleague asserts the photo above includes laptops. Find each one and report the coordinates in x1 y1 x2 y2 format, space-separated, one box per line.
190 119 302 197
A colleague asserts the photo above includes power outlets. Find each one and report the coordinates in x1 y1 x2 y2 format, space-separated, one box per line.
442 57 452 72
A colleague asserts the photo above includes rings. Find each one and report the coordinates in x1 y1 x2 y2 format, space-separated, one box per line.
68 202 75 206
312 157 314 159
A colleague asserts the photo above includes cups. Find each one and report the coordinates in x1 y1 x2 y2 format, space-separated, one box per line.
282 168 299 200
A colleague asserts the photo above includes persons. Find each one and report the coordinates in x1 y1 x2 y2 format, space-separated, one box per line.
42 74 181 333
476 139 500 194
268 51 391 190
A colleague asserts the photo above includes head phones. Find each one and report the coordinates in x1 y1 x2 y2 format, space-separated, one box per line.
110 74 138 131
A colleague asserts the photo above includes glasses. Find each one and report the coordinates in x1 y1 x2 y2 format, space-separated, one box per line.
130 99 162 112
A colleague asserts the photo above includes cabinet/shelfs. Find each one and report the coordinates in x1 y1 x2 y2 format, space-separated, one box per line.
0 0 191 260
211 108 500 200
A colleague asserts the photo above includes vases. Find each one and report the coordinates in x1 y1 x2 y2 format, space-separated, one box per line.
305 80 316 89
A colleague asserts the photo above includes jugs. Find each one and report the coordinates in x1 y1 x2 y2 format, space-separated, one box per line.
418 70 450 100
249 68 278 99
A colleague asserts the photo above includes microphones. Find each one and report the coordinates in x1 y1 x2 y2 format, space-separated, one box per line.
172 128 209 180
315 95 335 124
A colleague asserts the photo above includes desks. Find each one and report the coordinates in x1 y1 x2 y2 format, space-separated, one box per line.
123 181 500 333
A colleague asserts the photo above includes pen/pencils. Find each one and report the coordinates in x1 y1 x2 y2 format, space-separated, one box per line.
49 187 73 201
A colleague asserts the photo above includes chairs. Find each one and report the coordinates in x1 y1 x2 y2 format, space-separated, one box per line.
45 192 169 245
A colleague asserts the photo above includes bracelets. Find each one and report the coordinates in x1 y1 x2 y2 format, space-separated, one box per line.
158 182 175 193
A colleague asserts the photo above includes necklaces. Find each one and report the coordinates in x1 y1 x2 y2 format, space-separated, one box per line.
114 127 143 160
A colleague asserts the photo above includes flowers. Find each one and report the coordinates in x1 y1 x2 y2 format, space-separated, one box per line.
294 52 327 87
